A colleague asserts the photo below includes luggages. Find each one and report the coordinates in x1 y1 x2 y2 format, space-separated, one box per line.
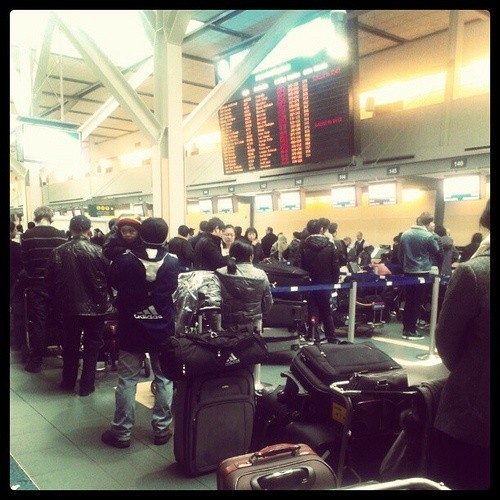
256 263 312 301
263 326 300 353
172 341 440 490
262 297 307 328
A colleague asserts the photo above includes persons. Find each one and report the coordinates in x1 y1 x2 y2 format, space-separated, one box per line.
418 225 459 331
215 237 274 395
398 212 442 341
102 213 145 263
434 195 491 490
16 207 81 374
50 215 109 397
11 212 405 352
100 217 180 450
459 232 482 263
294 219 340 345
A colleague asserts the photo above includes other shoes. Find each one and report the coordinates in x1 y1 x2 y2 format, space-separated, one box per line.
60 380 76 390
401 329 424 340
79 382 96 396
154 428 172 445
101 430 130 448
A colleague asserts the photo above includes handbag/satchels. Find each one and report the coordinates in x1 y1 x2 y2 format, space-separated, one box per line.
160 325 267 381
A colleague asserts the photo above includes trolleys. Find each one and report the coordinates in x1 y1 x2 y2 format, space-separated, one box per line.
77 305 151 378
320 381 435 490
9 292 61 363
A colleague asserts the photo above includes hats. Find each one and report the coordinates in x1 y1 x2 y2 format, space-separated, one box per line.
139 217 168 245
116 212 142 227
